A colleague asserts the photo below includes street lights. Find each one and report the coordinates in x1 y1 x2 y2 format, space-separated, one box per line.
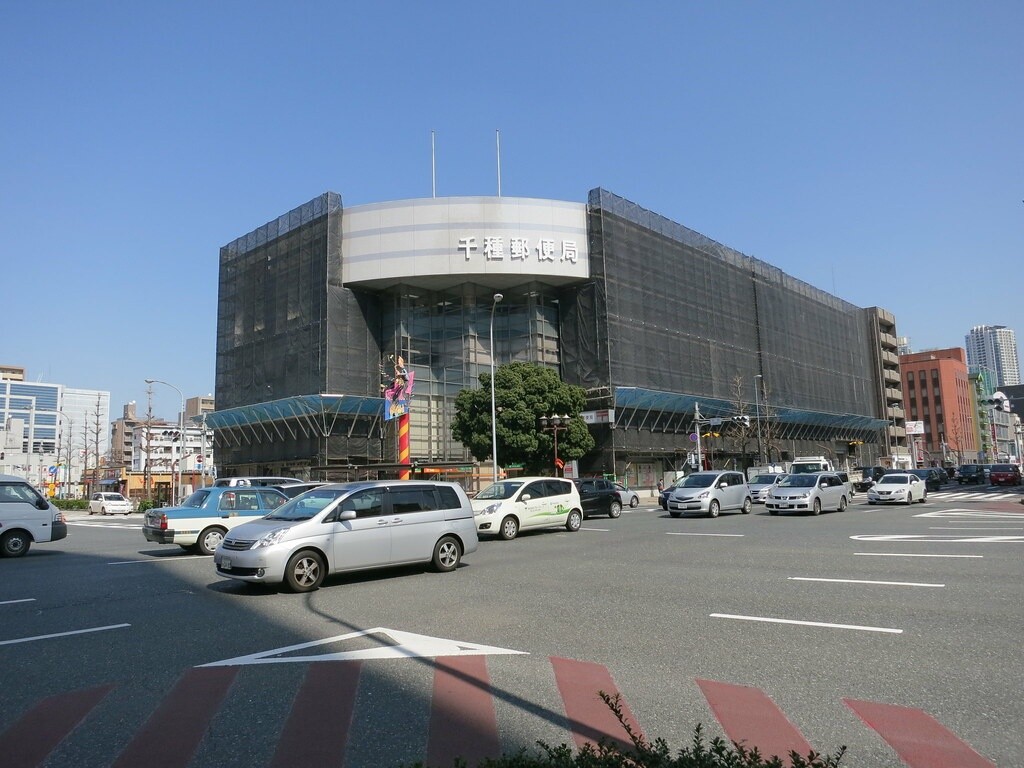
26 406 70 497
145 379 185 507
491 293 503 481
892 402 900 470
539 411 571 477
754 374 764 467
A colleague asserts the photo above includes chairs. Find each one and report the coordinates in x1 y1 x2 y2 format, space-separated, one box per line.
341 500 356 513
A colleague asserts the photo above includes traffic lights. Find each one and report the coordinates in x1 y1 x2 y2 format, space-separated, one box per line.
0 452 4 460
732 416 749 428
163 430 179 442
981 398 1003 412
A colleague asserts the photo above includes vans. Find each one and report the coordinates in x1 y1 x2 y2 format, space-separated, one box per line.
213 479 478 593
746 473 783 503
0 474 67 558
812 471 853 503
272 481 380 512
566 478 622 520
88 491 130 516
468 476 584 540
667 471 752 518
212 476 303 493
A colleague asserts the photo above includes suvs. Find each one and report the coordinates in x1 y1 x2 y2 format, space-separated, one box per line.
854 467 886 492
956 465 985 484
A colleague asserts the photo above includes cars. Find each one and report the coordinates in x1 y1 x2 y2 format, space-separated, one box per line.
142 487 322 556
989 464 1021 486
658 476 688 511
928 467 949 484
883 470 907 474
981 464 992 479
763 473 848 515
908 469 940 492
945 467 958 479
866 473 927 505
612 481 640 509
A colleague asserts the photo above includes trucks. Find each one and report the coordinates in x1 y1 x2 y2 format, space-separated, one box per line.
789 456 832 474
748 465 783 482
